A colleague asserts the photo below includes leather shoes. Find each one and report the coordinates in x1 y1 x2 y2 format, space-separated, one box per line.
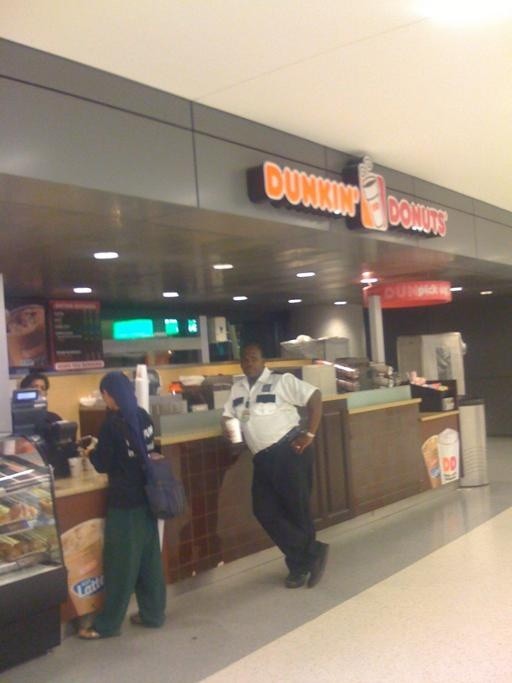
307 542 329 587
286 570 306 588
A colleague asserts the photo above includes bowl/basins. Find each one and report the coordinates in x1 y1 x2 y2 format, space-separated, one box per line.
180 375 205 385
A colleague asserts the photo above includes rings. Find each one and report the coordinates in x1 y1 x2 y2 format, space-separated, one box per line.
296 445 301 449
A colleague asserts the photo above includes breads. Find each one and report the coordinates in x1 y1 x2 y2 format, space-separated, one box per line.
0 503 38 524
0 540 43 557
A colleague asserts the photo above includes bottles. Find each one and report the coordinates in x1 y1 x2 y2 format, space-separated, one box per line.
170 382 183 400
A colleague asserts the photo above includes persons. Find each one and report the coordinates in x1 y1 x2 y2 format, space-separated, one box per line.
221 343 332 588
78 369 167 641
19 371 64 421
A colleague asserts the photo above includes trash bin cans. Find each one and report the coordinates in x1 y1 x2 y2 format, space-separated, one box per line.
458 399 487 487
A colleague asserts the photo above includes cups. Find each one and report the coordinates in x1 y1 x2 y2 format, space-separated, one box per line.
84 455 94 470
6 302 48 366
225 417 244 444
420 434 442 486
135 363 150 414
441 396 454 411
67 457 84 477
437 426 459 484
59 518 105 616
362 176 385 228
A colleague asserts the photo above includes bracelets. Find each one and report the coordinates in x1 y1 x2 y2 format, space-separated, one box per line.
304 431 315 438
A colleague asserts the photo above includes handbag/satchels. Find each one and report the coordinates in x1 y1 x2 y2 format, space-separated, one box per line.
141 452 185 519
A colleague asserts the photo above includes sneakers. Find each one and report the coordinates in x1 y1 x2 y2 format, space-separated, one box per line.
130 614 143 624
78 625 105 639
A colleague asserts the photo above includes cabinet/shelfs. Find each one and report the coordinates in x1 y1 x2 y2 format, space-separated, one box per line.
0 429 64 588
